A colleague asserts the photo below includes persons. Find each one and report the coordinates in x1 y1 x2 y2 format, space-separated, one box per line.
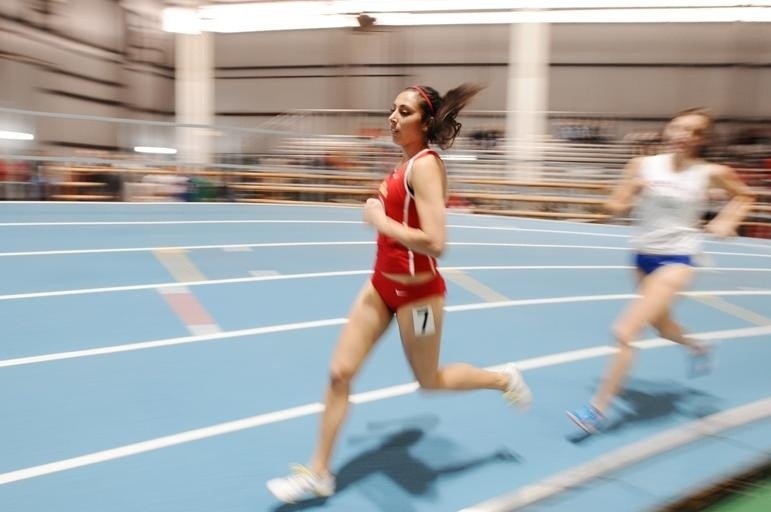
567 107 757 436
265 84 534 507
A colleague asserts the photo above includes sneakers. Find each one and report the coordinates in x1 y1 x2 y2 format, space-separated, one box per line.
265 470 336 505
501 364 529 405
